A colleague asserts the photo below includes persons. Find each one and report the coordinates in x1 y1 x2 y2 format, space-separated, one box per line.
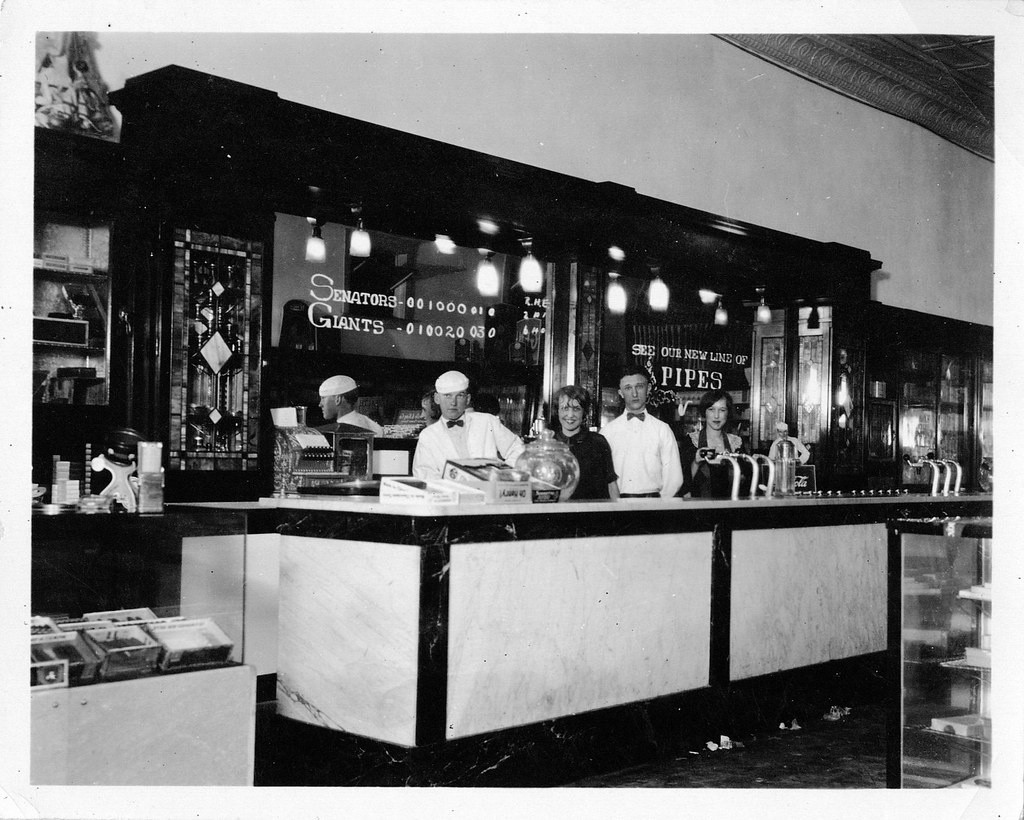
318 375 383 438
768 422 810 464
597 367 684 499
548 385 621 502
681 391 748 500
413 370 525 478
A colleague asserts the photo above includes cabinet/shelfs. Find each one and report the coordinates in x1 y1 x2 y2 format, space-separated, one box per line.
885 517 992 788
35 124 111 406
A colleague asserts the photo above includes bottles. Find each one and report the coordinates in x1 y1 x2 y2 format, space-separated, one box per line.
774 442 795 496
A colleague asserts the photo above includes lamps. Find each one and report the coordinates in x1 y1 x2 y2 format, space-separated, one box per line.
518 241 543 293
475 247 501 297
647 266 670 311
714 296 728 325
756 297 771 323
349 216 372 258
304 218 327 263
605 269 628 315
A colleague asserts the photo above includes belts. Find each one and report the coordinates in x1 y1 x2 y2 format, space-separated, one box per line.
619 492 661 498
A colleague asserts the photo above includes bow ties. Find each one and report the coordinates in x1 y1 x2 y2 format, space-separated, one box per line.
446 420 464 428
627 413 644 421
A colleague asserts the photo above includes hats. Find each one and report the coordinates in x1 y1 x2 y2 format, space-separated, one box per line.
318 375 357 398
436 371 470 394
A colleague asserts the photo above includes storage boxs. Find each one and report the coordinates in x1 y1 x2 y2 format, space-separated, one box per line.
380 457 561 507
30 608 233 693
